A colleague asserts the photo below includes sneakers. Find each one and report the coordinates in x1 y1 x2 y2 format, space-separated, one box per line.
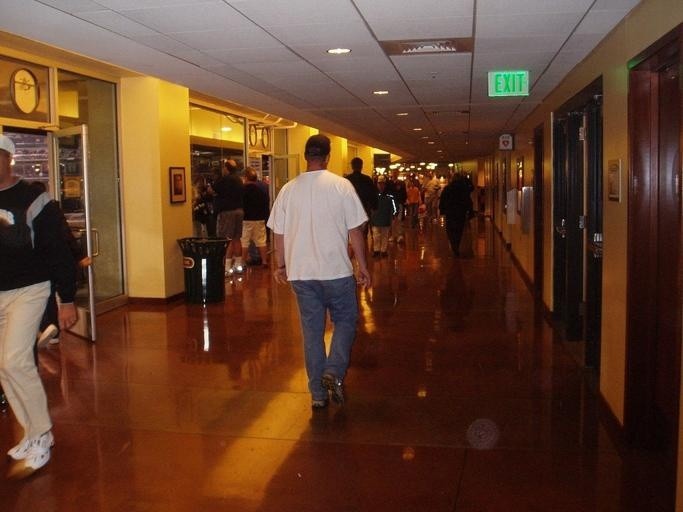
231 266 244 275
37 324 60 349
25 431 56 472
225 269 232 279
321 373 346 408
312 390 334 408
8 437 31 462
50 338 60 345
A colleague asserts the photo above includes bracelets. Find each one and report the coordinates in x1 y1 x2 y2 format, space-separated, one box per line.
277 266 284 269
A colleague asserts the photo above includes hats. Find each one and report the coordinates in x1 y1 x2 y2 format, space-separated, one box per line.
1 134 17 157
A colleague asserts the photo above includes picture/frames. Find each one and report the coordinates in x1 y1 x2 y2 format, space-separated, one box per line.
168 167 186 204
607 159 621 202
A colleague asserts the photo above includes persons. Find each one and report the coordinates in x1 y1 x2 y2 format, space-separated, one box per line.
345 157 492 258
265 134 372 411
191 158 269 276
1 134 83 482
31 181 91 350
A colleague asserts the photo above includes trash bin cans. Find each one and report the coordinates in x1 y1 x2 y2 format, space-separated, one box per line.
177 237 232 305
